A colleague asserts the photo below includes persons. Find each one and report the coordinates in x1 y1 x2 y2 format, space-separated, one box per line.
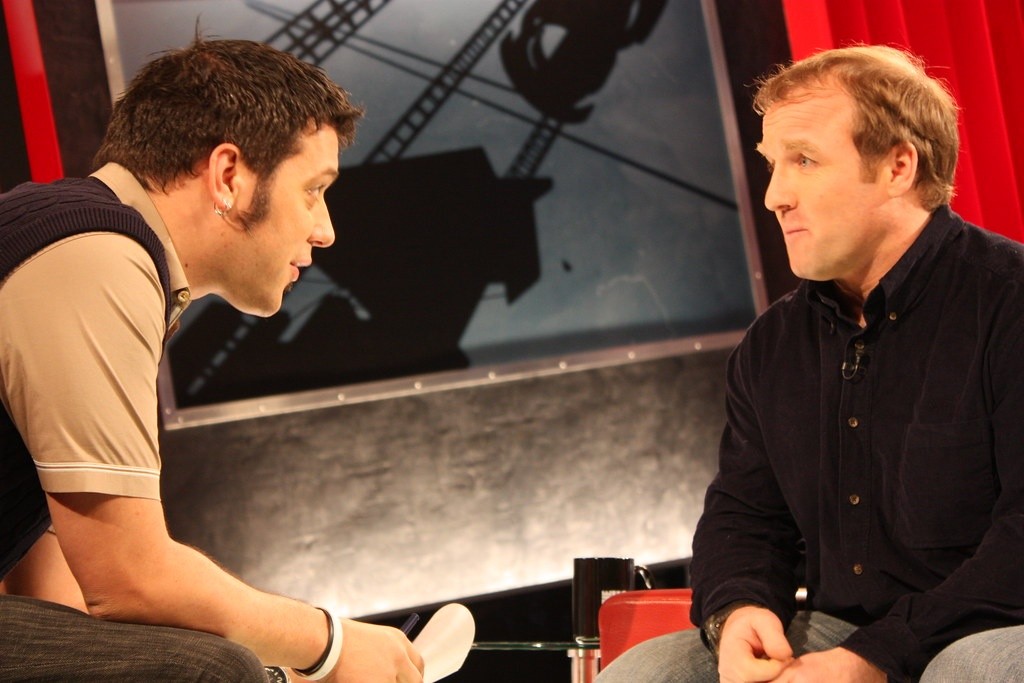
0 15 425 683
590 45 1024 682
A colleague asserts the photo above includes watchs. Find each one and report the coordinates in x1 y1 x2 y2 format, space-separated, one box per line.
704 600 751 665
264 665 294 683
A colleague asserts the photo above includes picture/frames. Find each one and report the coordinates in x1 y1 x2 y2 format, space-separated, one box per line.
95 0 770 434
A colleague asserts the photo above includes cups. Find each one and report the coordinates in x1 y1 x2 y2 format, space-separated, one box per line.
572 556 656 644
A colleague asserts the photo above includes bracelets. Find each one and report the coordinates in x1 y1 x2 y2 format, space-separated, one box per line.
292 606 343 681
